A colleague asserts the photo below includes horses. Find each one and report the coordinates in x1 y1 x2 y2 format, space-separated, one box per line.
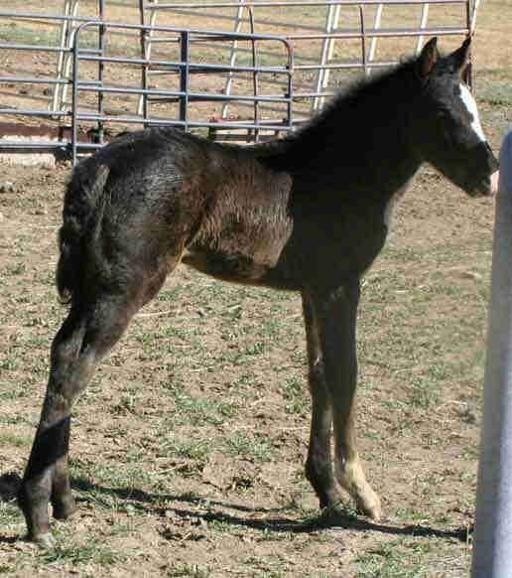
18 34 498 550
